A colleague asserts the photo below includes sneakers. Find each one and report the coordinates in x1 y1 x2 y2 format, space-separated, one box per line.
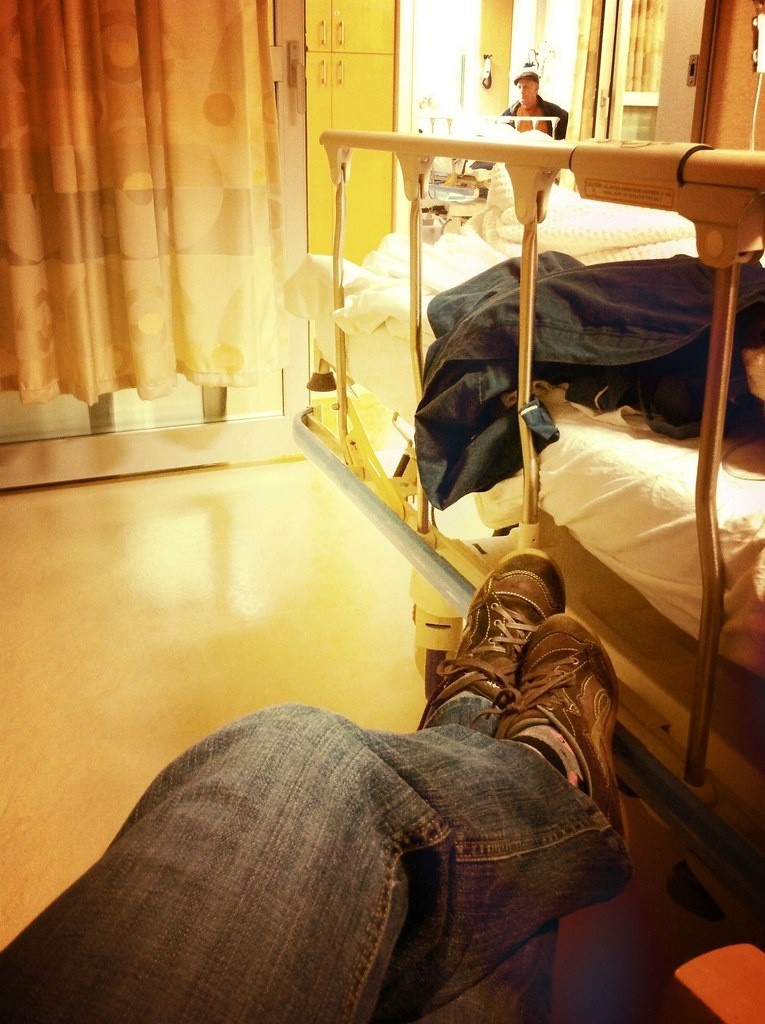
416 546 567 721
468 612 632 856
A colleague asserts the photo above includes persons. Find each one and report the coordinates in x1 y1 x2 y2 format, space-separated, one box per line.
498 70 569 140
3 549 638 1024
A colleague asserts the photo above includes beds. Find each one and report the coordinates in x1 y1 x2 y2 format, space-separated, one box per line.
421 109 565 217
299 126 765 885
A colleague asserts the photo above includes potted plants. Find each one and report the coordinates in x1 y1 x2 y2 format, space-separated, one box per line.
523 63 539 80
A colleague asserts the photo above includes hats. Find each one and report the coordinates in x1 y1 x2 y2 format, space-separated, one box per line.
514 71 539 85
